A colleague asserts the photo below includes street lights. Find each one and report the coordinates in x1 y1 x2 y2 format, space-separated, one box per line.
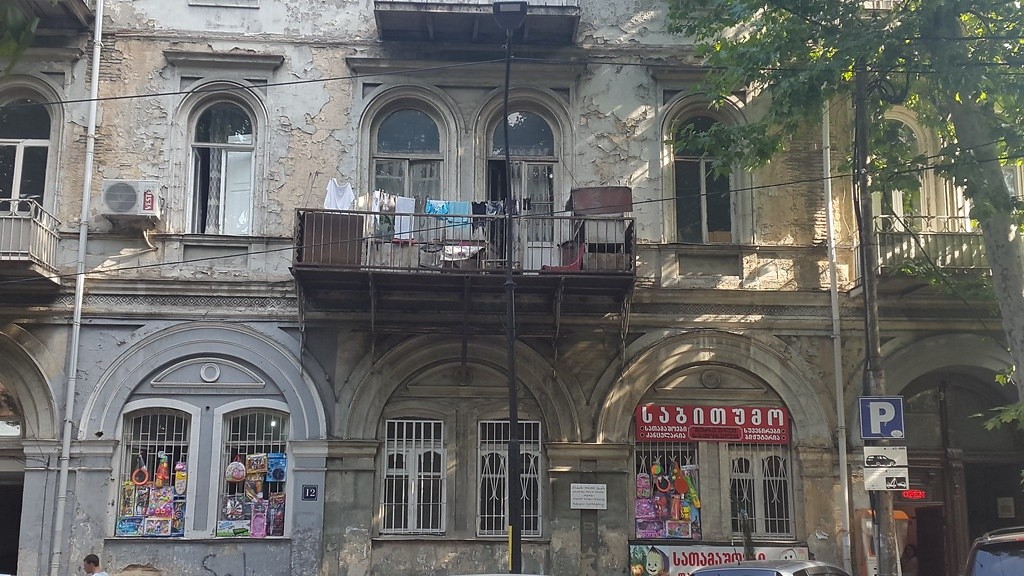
494 1 533 576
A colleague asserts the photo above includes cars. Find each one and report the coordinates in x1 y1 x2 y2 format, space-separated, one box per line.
687 559 850 576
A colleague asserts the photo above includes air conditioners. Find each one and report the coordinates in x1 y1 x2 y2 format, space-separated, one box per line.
100 177 162 222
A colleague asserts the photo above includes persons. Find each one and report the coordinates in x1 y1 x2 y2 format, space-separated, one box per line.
901 544 919 576
83 554 110 576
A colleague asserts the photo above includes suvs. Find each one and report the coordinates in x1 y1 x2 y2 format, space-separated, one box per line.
964 526 1024 576
